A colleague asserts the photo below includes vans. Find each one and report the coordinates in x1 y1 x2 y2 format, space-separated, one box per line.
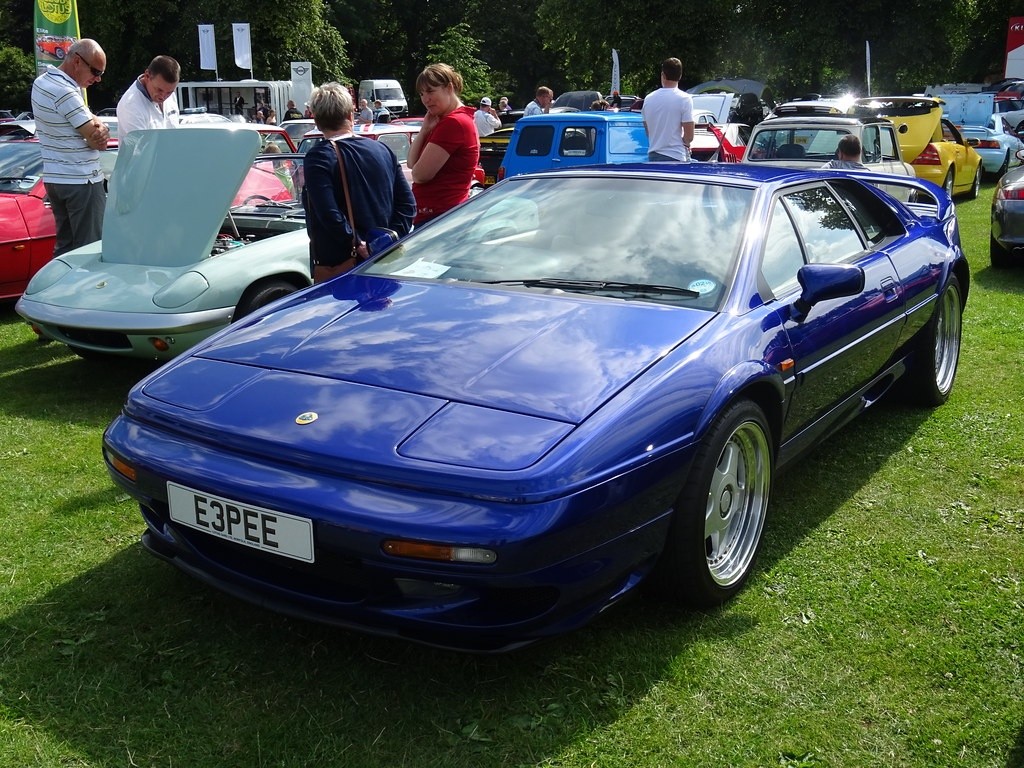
359 79 408 122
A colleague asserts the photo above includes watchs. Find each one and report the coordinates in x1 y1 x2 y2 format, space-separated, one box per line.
103 122 110 131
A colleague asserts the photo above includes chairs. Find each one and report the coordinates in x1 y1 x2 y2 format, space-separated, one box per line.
835 146 869 162
553 191 644 262
565 136 591 157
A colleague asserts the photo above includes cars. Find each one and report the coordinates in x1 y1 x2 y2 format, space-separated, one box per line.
932 92 1024 185
353 78 770 226
989 148 1024 275
739 116 919 205
99 162 972 645
15 125 414 374
859 95 983 201
0 105 315 301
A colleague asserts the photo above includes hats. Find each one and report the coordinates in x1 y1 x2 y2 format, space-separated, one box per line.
614 92 618 95
480 97 491 106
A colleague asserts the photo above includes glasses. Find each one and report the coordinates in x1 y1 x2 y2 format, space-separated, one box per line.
75 52 105 77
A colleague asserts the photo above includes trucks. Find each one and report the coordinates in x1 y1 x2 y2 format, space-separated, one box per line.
924 83 990 97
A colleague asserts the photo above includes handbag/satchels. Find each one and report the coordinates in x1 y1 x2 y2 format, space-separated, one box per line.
314 259 355 284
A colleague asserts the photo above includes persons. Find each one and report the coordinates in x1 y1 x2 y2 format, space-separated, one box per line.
523 86 554 117
640 58 695 162
116 56 181 151
302 82 418 279
407 62 481 232
31 38 111 342
819 135 873 172
262 143 296 199
591 91 621 111
235 91 390 138
474 97 512 137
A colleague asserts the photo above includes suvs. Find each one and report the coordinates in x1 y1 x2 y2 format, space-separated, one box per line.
762 94 859 120
987 77 1024 134
